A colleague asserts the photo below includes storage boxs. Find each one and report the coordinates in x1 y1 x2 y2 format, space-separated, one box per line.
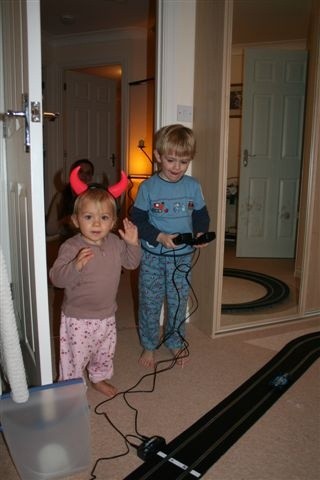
0 377 92 480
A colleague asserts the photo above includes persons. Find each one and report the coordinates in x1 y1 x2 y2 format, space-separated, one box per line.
48 165 143 398
127 123 211 367
47 159 95 244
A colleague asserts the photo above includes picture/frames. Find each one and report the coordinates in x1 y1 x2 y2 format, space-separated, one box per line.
229 83 243 118
